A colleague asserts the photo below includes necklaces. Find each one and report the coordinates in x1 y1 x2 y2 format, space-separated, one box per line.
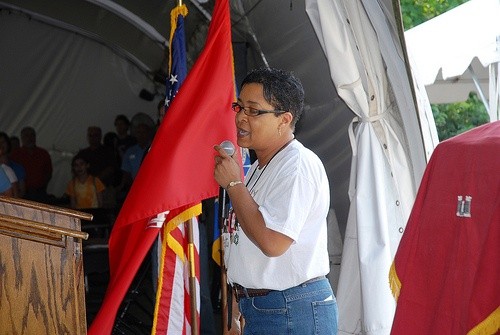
226 138 294 232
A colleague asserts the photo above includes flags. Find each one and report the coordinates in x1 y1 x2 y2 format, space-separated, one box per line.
87 0 245 335
150 4 200 335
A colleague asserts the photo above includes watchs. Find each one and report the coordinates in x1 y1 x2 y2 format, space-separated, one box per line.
227 181 242 188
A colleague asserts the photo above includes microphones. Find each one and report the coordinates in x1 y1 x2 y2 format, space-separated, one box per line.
219 140 235 218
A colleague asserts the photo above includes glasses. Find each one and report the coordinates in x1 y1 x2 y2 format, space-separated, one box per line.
231 102 287 117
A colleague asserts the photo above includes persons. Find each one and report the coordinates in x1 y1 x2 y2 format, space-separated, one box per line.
65 112 156 285
0 126 53 203
151 95 217 335
213 67 340 335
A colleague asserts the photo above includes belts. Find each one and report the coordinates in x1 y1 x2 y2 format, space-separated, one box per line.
232 275 326 303
